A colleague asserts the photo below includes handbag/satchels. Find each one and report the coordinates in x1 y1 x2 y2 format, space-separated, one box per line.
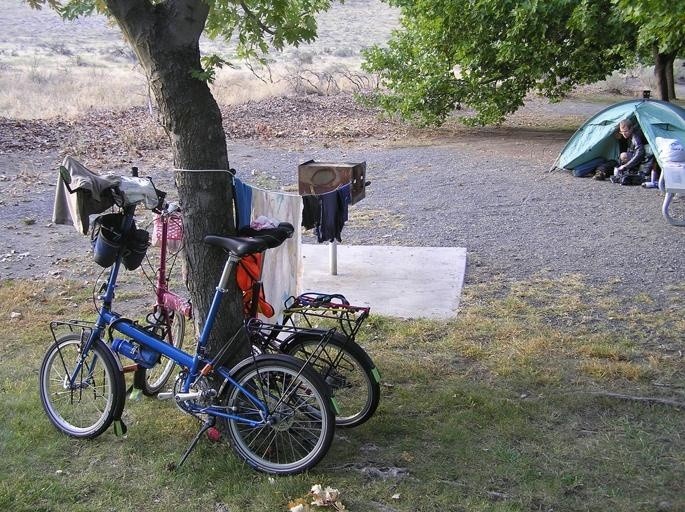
620 168 644 184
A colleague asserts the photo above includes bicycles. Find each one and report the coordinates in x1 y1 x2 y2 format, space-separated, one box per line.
39 154 335 477
128 165 381 430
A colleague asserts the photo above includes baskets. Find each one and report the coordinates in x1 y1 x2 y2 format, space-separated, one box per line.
153 214 183 250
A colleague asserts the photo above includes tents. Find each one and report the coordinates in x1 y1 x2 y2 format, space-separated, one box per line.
548 96 685 174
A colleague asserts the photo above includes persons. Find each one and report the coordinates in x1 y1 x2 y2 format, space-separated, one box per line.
613 119 646 174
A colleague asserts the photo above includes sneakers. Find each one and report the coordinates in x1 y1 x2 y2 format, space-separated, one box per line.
592 171 606 180
